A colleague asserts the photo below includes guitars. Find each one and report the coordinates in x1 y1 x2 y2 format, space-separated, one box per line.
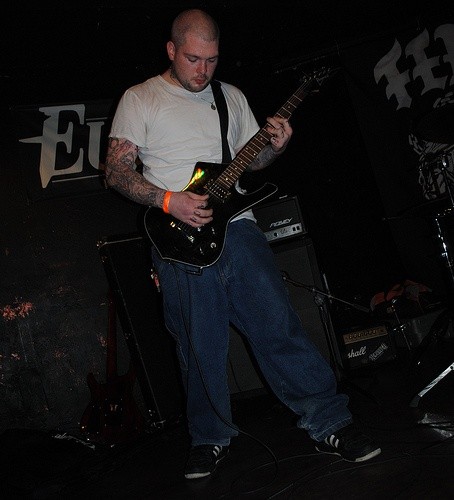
79 288 147 440
144 65 334 276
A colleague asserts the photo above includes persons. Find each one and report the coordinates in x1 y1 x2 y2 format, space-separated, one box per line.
105 9 381 478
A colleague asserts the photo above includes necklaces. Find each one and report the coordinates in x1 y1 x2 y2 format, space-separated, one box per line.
170 76 217 111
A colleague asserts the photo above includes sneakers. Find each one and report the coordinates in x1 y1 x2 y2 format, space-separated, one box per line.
184 444 229 478
314 430 381 463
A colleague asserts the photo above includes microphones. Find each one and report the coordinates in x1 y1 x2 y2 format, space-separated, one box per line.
279 270 290 280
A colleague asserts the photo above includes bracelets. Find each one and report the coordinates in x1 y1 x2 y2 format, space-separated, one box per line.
162 191 172 214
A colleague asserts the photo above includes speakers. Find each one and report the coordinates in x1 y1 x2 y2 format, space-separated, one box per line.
97 231 189 427
226 238 346 402
